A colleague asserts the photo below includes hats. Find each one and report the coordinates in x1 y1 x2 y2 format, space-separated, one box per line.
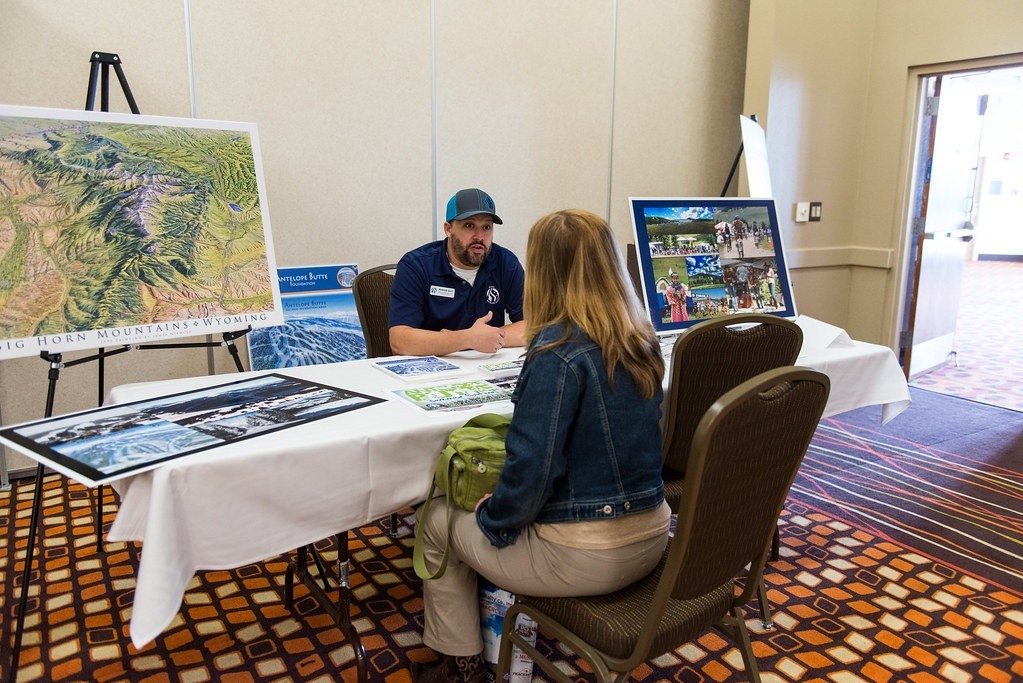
445 187 503 224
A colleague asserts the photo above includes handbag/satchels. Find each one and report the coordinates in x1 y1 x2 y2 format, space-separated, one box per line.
433 413 511 511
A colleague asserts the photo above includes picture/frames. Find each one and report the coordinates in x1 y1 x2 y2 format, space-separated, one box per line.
628 198 798 335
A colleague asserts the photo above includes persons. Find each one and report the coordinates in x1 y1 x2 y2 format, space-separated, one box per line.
386 189 524 358
414 209 672 683
649 215 780 323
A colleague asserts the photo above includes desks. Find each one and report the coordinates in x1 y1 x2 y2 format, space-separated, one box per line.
100 322 914 682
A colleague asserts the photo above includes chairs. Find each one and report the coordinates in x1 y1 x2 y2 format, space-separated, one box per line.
495 367 831 683
656 315 804 630
353 263 406 359
